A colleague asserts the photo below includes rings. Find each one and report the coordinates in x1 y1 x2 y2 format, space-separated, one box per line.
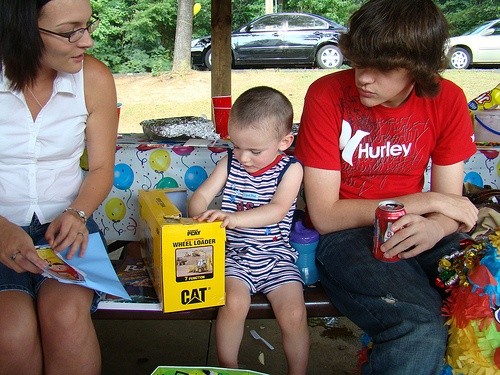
11 253 17 261
78 232 84 237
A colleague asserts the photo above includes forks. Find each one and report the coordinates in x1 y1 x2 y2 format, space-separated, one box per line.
250 330 274 350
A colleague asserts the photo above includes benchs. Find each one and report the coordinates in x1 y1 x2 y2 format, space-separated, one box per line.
80 133 500 321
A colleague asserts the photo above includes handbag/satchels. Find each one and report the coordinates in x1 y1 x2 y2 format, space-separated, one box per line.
463 182 500 239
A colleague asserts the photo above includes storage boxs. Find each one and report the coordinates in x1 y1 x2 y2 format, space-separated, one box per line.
473 110 500 143
137 189 226 313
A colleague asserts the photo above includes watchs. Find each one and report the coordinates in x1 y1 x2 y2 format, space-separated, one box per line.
63 208 88 224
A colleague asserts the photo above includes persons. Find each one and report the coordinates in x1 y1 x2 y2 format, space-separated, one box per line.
188 86 311 375
0 0 118 375
295 0 479 375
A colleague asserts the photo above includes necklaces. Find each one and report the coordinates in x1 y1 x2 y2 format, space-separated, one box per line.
27 87 44 108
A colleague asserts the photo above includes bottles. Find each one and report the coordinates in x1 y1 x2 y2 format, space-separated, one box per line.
290 222 321 285
468 83 500 115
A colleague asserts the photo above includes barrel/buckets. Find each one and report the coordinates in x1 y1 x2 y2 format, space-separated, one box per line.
473 110 500 142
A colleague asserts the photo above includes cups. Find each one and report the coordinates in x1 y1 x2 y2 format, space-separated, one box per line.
117 103 123 122
212 96 232 108
213 108 232 139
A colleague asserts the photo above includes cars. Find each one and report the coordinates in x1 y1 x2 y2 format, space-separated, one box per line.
190 10 350 71
442 18 500 70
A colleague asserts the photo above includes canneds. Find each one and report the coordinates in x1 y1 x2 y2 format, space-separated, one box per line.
373 200 407 262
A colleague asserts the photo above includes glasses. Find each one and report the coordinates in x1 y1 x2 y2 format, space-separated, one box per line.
38 15 100 43
